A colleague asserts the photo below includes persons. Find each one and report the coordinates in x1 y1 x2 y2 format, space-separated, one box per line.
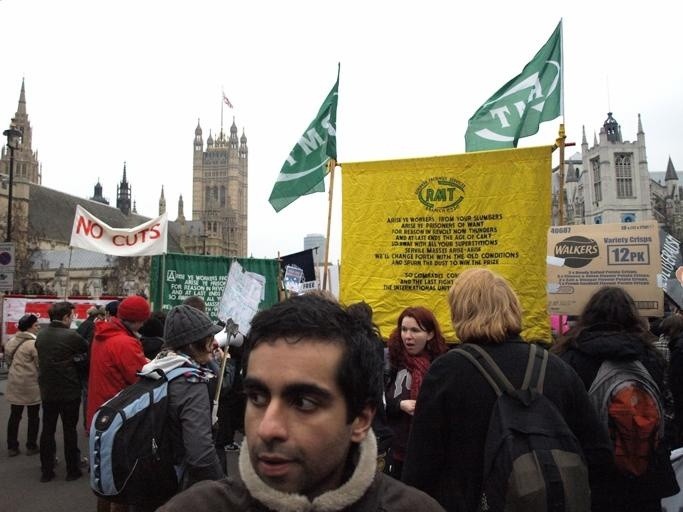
549 286 681 512
84 294 152 512
181 296 240 452
382 305 450 478
34 301 91 483
75 304 105 437
658 312 683 447
399 267 615 512
105 300 121 317
346 300 382 345
154 294 447 512
141 304 228 512
3 313 41 458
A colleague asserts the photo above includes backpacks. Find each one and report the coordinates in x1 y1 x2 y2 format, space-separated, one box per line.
88 365 205 506
587 357 665 481
444 338 593 512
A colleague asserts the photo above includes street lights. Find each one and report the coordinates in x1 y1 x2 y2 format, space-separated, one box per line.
3 118 23 243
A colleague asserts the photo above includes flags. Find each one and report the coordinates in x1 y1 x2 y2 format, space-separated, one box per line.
268 69 341 214
463 17 565 154
223 93 234 109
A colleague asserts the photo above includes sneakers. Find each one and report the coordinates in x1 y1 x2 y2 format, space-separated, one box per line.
80 457 88 467
26 445 40 455
223 443 240 453
7 446 20 457
66 472 81 479
40 472 55 483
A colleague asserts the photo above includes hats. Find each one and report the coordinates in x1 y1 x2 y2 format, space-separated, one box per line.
17 314 37 332
161 305 223 352
116 296 151 322
